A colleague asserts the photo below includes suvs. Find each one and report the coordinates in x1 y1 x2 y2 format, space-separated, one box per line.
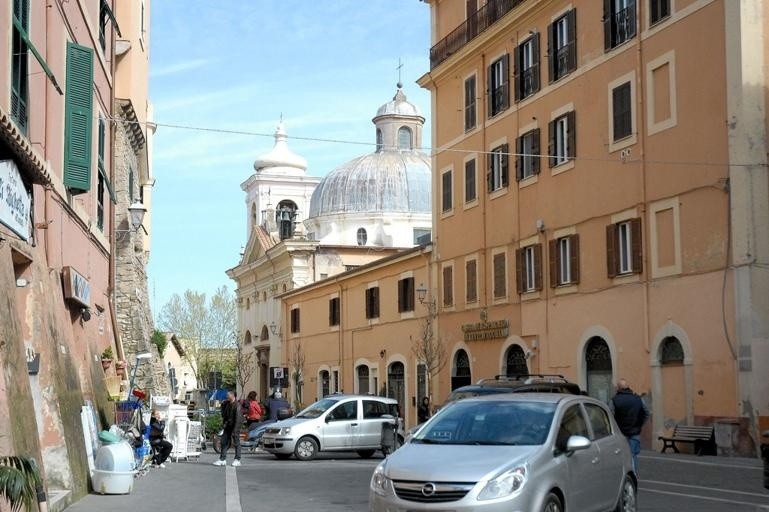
401 371 591 443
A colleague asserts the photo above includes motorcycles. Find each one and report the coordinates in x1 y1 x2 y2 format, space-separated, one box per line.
211 391 291 456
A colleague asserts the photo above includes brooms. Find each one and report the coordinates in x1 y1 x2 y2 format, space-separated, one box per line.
123 390 149 435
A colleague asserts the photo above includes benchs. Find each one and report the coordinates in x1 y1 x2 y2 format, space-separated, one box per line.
658 425 715 457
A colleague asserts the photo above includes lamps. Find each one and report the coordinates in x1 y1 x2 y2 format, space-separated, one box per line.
269 321 281 335
416 283 436 305
380 349 385 358
114 198 147 243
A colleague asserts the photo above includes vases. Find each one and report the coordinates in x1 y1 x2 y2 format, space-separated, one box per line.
117 367 124 375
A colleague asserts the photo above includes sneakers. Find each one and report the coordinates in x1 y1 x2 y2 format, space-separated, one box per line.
231 458 241 467
152 463 166 469
212 458 227 466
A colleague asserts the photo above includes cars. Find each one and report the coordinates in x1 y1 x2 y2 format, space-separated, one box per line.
258 392 406 461
365 390 642 512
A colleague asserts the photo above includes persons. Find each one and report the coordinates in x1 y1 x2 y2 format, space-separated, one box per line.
605 377 650 478
149 408 174 469
246 390 264 452
416 396 432 424
212 390 245 468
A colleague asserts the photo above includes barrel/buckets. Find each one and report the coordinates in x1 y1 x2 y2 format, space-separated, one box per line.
95 441 136 472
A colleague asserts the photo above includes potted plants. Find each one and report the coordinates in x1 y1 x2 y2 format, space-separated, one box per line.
101 346 112 370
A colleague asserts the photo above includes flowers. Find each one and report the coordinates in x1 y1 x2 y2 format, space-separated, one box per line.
115 361 127 369
